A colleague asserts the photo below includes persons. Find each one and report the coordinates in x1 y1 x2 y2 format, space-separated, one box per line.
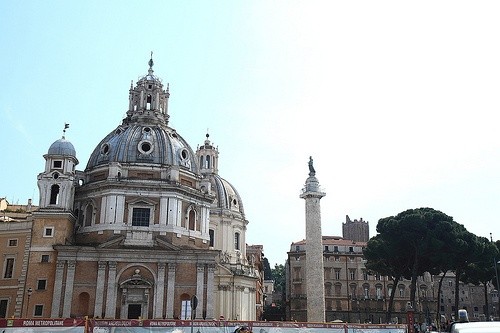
364 316 494 333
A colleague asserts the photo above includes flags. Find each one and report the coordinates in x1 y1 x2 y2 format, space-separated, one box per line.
65 124 69 128
